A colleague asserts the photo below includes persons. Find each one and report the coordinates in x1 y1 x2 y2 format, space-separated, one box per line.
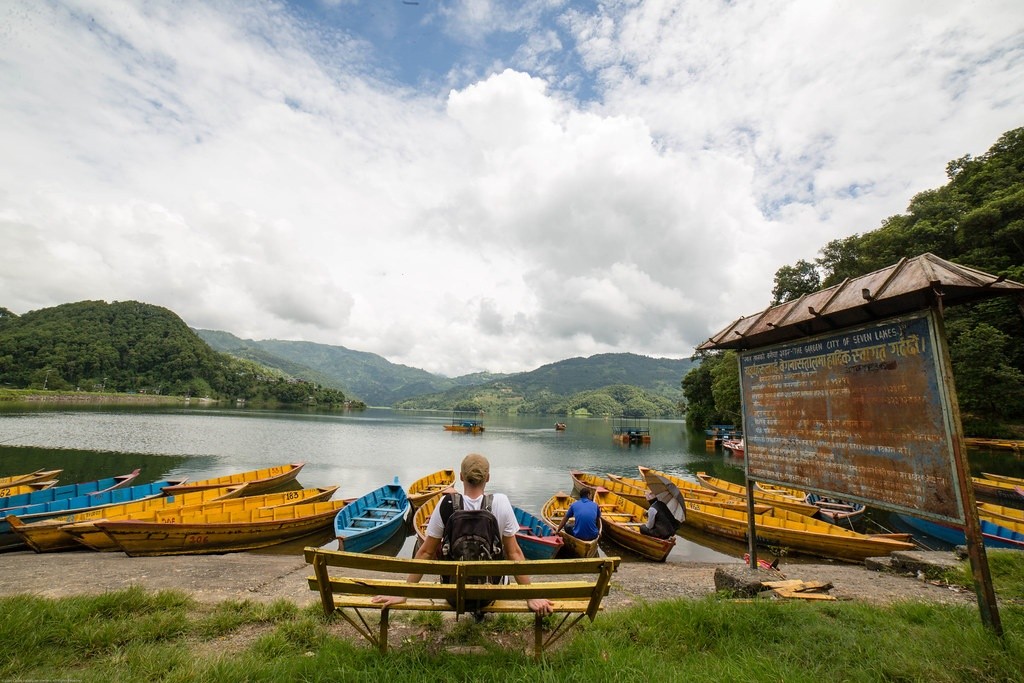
555 488 601 541
371 454 554 618
640 492 680 539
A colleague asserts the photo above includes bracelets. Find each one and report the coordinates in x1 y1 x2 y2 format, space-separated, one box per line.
556 528 561 533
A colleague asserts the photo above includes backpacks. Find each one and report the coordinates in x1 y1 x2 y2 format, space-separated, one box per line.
441 492 503 613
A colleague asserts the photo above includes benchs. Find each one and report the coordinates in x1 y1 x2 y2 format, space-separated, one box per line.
601 513 636 517
767 490 787 493
350 517 389 522
420 524 429 526
599 503 619 508
518 525 532 531
300 545 622 662
363 507 401 512
426 515 431 518
553 509 568 512
550 516 575 521
820 508 848 514
419 490 433 493
380 498 399 502
813 500 855 509
428 484 447 488
614 522 646 526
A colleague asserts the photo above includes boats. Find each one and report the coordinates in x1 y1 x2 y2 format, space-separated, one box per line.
7 482 250 555
569 467 915 561
92 499 355 556
409 468 456 507
540 490 600 559
412 487 459 549
722 436 744 455
593 486 677 562
334 476 410 552
160 462 304 495
0 469 140 511
0 480 59 497
0 468 64 489
56 485 339 551
895 471 1024 550
444 408 484 432
0 476 190 540
555 421 566 431
511 506 563 560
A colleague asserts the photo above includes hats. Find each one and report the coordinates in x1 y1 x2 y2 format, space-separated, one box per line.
645 491 657 501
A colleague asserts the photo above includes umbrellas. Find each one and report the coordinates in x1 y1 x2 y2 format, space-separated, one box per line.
645 472 686 523
